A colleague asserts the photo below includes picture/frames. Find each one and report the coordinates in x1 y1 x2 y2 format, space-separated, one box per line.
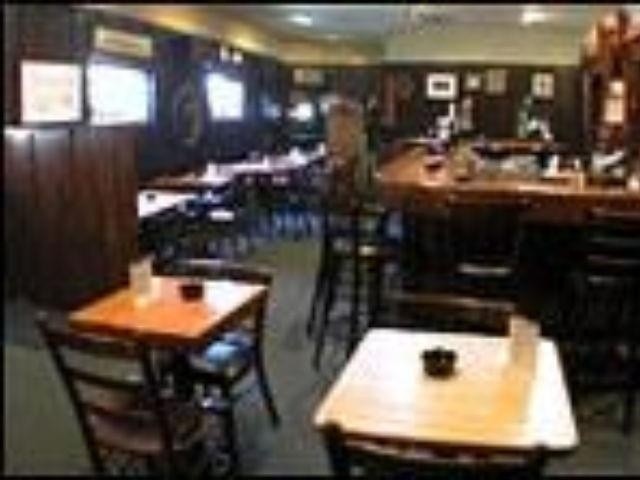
427 73 458 100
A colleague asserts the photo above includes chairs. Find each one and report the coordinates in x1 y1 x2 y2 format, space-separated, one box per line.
573 223 638 337
314 205 409 372
449 197 527 297
378 294 512 337
322 422 545 480
306 196 396 340
36 318 209 478
158 263 283 428
